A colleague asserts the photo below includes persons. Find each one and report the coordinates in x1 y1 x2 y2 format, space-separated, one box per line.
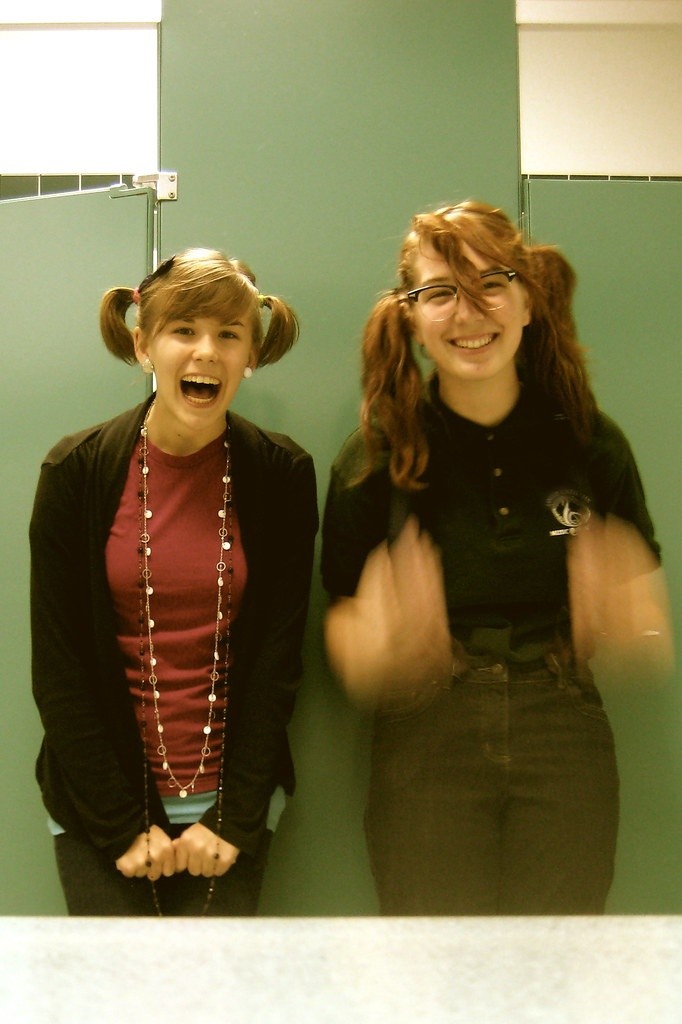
27 245 318 917
316 202 673 917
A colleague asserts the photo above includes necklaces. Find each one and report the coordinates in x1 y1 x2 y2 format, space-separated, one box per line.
135 401 236 916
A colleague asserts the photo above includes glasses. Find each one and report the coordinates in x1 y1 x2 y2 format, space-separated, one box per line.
407 268 516 323
